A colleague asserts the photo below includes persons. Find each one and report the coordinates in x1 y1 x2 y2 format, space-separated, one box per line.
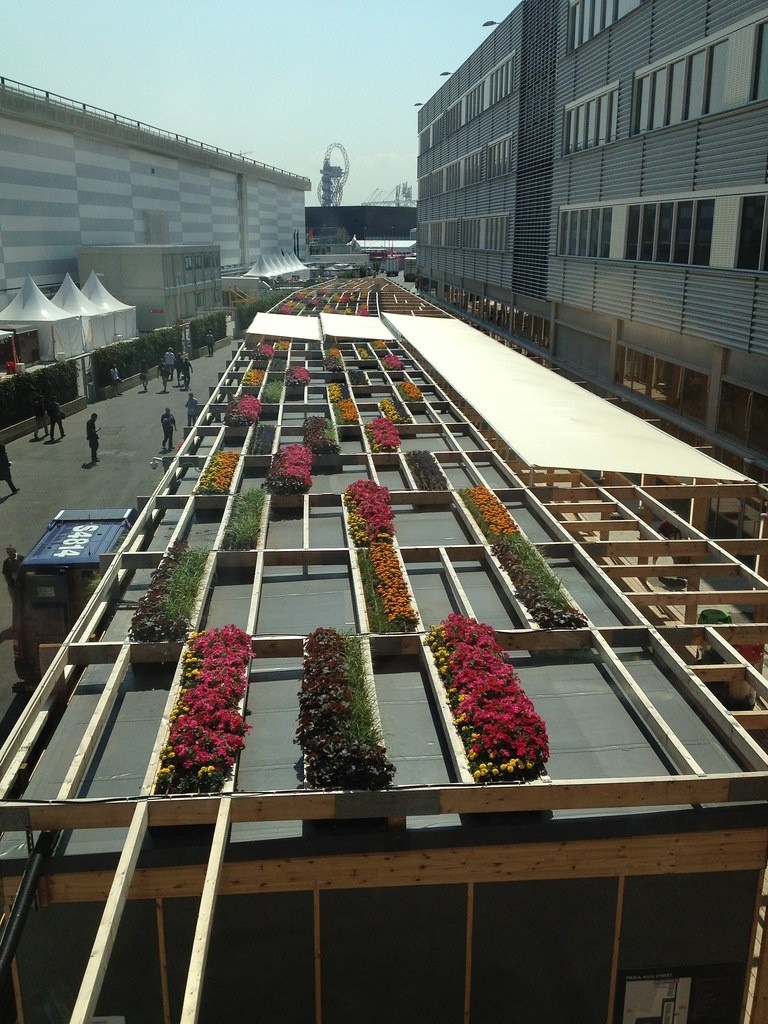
110 364 124 395
34 395 50 439
160 408 178 450
47 396 66 442
185 393 197 426
0 443 20 494
86 412 102 462
156 347 194 392
2 544 26 601
206 331 215 356
139 360 149 392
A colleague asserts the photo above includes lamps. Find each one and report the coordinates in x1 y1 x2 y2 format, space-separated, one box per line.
483 20 502 26
440 72 454 76
414 103 426 106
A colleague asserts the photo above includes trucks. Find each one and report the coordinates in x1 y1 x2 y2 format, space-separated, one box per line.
404 257 417 282
385 258 399 277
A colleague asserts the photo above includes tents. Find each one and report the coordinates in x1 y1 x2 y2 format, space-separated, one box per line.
243 251 310 283
0 270 138 363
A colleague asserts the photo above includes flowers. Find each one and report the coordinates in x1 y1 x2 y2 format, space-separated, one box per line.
132 275 577 790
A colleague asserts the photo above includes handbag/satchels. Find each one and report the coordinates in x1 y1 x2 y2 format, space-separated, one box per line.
59 410 65 419
139 373 148 380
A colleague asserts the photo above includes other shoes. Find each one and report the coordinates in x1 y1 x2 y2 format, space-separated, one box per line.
51 437 54 441
34 435 39 441
61 433 66 438
92 459 100 463
45 434 50 436
163 440 165 446
169 446 175 449
12 488 20 494
91 456 98 459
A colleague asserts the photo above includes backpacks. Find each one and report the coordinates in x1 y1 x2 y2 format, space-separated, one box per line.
31 400 42 416
47 404 59 416
